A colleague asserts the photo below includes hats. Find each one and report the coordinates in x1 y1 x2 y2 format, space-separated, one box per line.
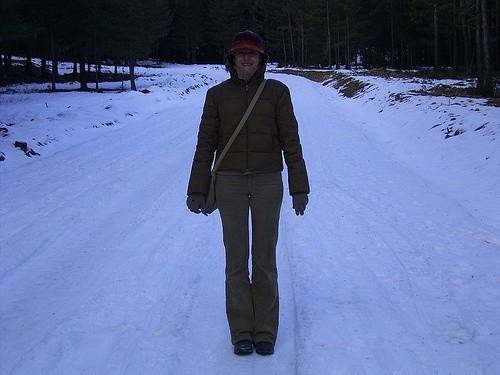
225 31 269 64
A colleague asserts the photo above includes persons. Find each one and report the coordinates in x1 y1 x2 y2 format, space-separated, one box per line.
185 30 311 356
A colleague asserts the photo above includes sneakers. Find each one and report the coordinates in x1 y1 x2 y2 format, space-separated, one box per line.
256 342 274 356
234 340 253 354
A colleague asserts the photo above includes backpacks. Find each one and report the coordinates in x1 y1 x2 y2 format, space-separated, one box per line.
204 161 218 215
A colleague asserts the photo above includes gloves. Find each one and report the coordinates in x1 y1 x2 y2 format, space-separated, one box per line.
291 193 310 215
187 193 207 214
